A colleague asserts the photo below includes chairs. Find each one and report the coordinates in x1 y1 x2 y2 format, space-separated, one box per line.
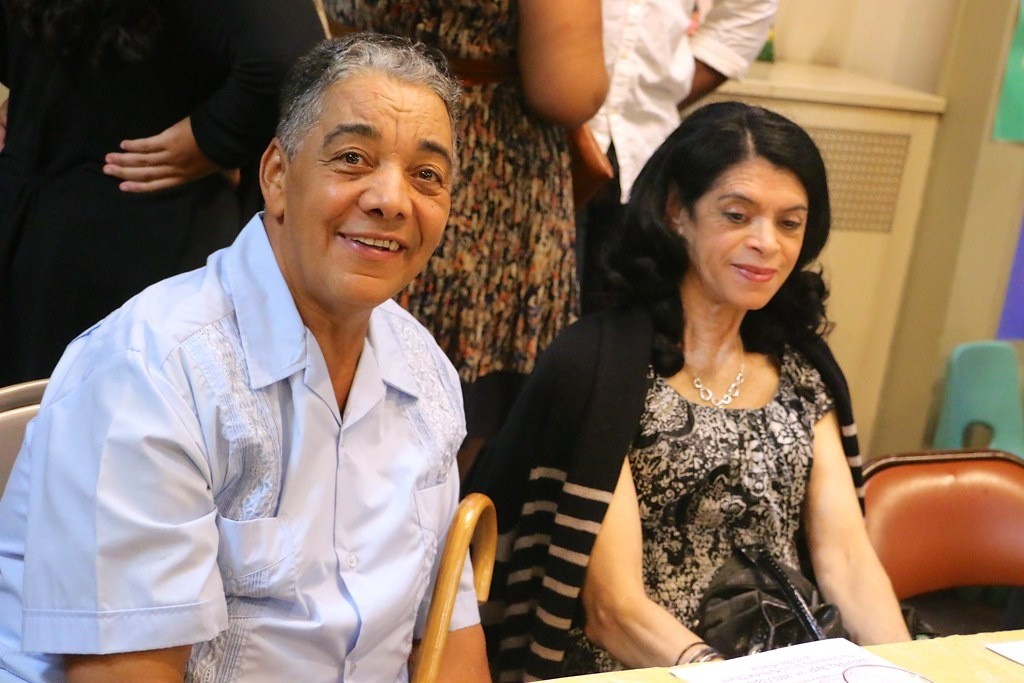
856 449 1024 641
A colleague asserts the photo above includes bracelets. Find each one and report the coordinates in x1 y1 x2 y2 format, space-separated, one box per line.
674 642 729 666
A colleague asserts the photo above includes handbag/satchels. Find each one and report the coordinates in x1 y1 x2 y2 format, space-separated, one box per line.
692 542 918 661
570 122 615 218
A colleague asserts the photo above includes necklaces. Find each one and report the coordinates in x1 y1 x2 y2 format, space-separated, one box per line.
683 360 747 409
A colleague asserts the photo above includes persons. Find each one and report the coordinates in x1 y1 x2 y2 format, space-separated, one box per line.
321 0 781 489
0 31 493 683
461 100 914 683
0 0 327 388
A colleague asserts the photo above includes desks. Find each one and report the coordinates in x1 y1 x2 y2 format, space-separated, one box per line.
686 59 949 466
